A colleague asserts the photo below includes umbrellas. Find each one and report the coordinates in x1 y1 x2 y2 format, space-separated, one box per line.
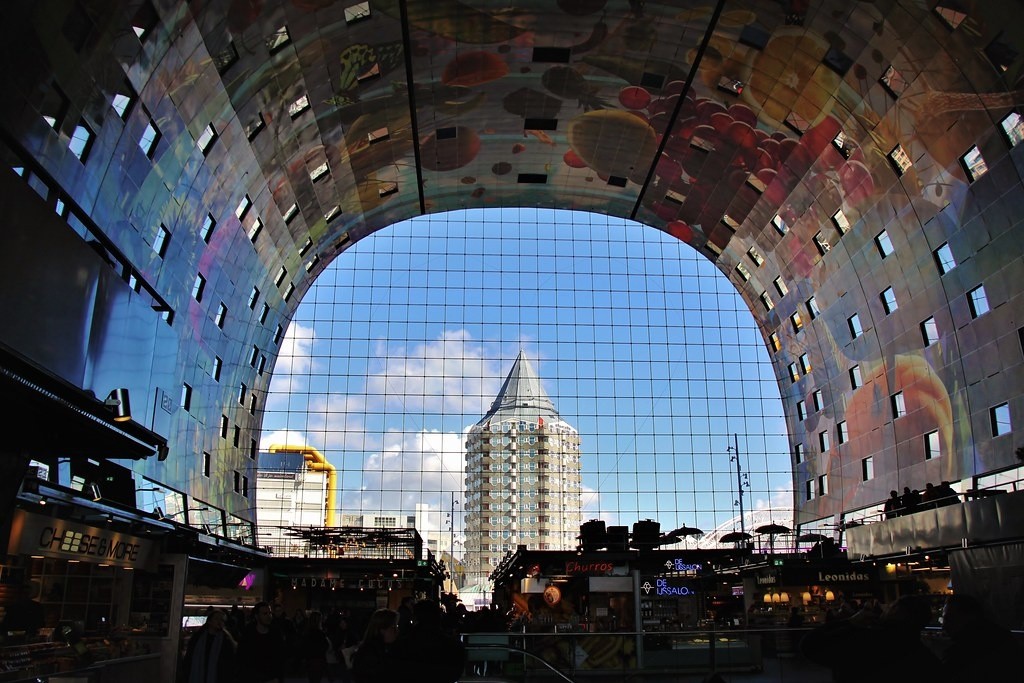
755 523 792 555
719 529 751 550
654 532 682 551
666 521 703 552
798 533 828 553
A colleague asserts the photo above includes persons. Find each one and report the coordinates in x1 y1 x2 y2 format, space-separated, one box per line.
800 591 949 683
885 480 960 518
301 611 330 682
333 615 359 682
180 609 237 682
938 591 1024 683
786 605 807 661
396 594 416 635
459 603 513 678
205 601 306 661
349 607 400 683
235 601 284 683
385 597 469 682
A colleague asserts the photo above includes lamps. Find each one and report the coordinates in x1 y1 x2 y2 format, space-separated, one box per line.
41 483 272 555
763 556 929 603
104 388 133 422
